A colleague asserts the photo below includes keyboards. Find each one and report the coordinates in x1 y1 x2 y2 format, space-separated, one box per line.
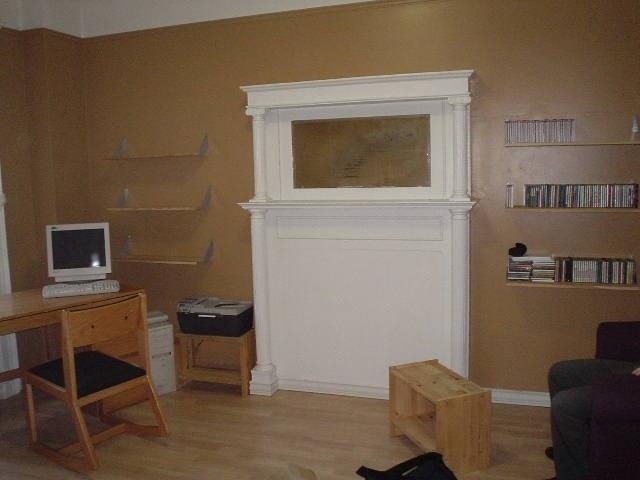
42 279 122 300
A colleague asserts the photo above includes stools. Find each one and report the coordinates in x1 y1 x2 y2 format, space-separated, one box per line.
179 330 255 396
386 359 491 473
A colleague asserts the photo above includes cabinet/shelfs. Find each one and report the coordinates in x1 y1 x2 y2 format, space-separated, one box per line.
101 132 216 268
502 138 640 291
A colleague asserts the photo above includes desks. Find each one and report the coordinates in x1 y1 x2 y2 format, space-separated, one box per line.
0 282 151 416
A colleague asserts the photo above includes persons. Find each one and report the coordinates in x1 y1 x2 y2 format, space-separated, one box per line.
542 354 640 480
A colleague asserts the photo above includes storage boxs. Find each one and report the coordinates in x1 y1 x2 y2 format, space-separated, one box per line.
176 296 256 337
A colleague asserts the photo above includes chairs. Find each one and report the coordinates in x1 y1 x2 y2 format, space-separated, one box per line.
19 291 174 472
591 320 639 479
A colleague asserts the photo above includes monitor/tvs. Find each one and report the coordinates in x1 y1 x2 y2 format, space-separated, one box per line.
45 222 113 284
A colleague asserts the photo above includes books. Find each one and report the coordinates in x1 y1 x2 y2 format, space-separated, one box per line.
504 117 575 145
504 183 639 209
503 254 637 287
